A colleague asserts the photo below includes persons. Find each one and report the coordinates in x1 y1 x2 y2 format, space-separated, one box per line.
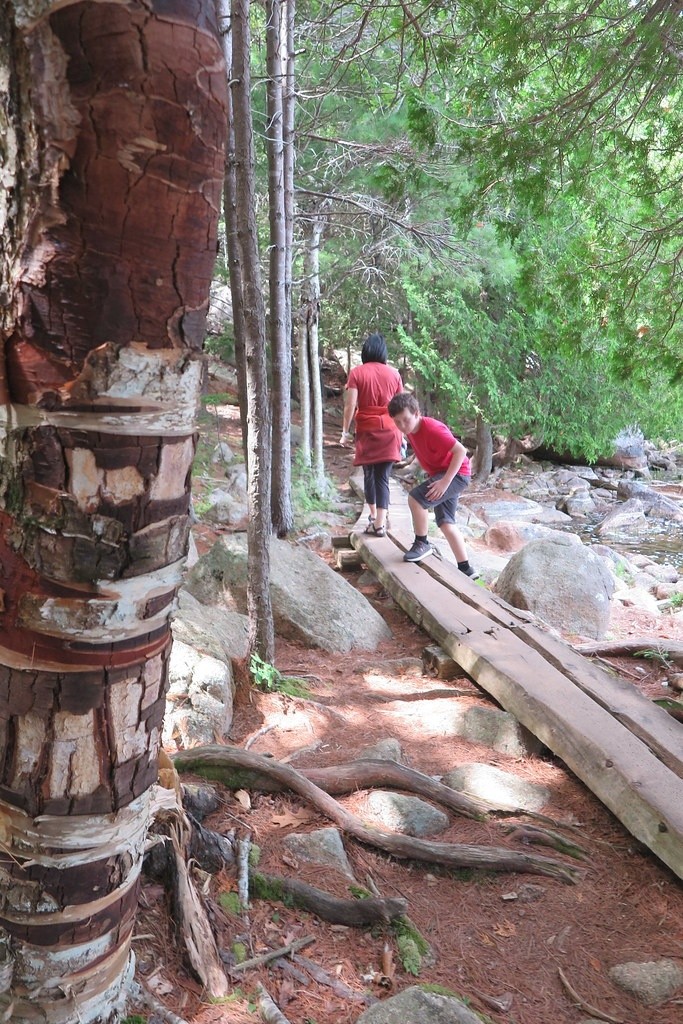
339 334 404 537
388 393 479 580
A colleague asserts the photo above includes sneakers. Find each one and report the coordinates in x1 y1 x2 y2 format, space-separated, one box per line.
403 541 432 561
464 567 479 579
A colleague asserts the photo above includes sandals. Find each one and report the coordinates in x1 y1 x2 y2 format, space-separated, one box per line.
364 524 386 537
368 514 376 521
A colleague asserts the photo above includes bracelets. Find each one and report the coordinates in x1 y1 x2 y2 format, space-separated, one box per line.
342 432 349 437
